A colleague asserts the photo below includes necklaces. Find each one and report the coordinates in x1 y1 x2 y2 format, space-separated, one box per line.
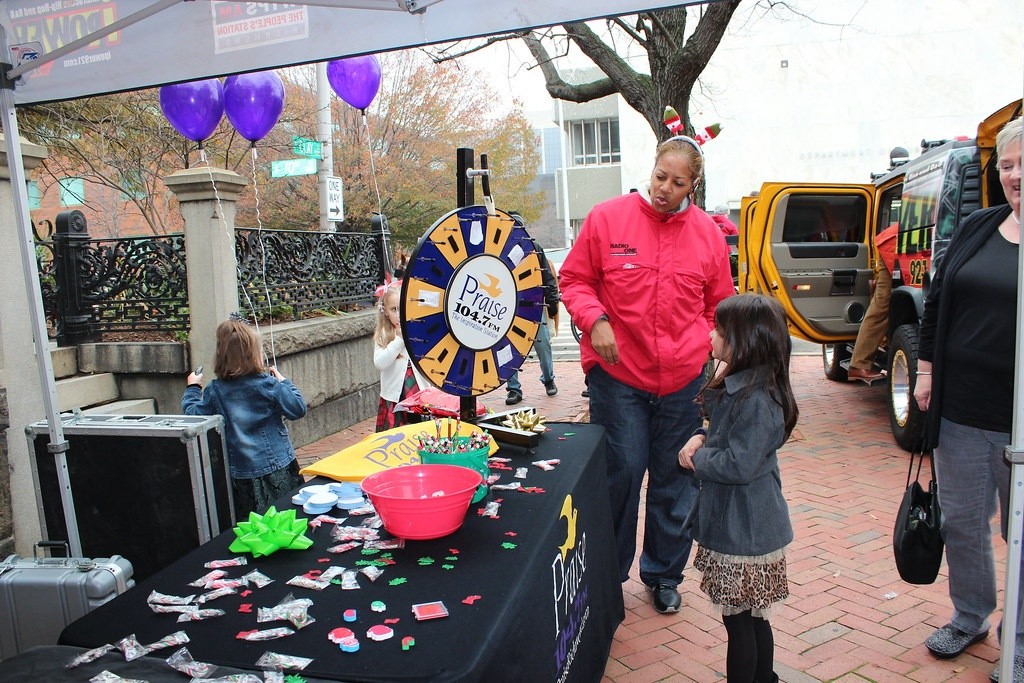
1012 210 1020 225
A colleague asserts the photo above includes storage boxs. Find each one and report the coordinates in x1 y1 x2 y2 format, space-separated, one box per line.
25 412 236 584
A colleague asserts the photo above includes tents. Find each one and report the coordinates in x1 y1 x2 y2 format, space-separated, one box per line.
0 0 709 558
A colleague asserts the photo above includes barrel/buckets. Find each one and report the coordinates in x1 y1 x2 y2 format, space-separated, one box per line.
417 437 490 503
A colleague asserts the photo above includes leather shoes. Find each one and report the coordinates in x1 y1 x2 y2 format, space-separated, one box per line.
847 367 885 381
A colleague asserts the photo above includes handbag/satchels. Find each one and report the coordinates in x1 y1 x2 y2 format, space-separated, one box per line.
893 405 945 585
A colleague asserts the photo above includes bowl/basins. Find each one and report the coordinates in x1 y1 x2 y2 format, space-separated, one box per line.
359 463 483 541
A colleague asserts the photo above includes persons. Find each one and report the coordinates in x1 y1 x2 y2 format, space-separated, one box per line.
180 321 307 524
678 294 799 683
913 116 1024 683
374 281 434 434
848 223 898 381
558 135 737 612
505 211 560 405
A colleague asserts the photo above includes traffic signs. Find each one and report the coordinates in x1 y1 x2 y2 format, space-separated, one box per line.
325 176 345 223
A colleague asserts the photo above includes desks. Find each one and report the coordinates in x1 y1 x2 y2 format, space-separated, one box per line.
0 645 343 683
58 422 625 683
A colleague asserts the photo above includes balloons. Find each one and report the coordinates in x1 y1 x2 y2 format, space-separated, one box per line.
159 78 225 150
326 54 381 116
224 70 285 147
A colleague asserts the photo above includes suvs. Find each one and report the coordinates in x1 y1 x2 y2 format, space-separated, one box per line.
736 95 1022 455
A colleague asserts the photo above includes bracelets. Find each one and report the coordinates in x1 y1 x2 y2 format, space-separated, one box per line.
916 372 932 375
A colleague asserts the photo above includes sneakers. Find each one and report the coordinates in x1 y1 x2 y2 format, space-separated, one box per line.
645 582 681 613
924 621 989 657
544 378 558 395
505 390 523 405
988 652 1023 683
